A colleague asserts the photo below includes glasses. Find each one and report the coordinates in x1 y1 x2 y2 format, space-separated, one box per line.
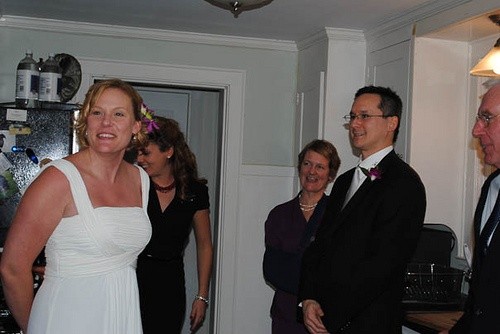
342 113 395 122
475 112 500 128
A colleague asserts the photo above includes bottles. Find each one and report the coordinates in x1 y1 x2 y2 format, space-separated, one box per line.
37 51 62 103
14 49 37 104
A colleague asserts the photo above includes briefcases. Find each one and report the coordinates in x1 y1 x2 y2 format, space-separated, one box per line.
403 224 466 304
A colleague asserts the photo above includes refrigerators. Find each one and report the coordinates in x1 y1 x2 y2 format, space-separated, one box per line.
0 102 83 334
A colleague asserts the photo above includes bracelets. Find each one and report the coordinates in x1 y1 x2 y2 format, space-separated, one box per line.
194 295 210 307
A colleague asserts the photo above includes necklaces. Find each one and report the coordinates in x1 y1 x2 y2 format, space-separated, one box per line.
151 180 176 194
298 192 318 212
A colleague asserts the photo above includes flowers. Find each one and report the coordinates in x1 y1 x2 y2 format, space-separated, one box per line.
141 103 159 132
368 167 382 181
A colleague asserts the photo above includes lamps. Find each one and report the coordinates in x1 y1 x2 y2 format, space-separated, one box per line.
468 38 500 79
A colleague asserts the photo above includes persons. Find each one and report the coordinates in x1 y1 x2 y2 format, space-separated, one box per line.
263 139 341 334
0 79 152 334
427 83 500 334
297 85 426 334
131 115 213 334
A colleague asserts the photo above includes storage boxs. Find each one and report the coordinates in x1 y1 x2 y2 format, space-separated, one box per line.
403 263 464 300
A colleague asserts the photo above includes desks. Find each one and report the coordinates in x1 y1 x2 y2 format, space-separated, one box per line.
406 312 465 331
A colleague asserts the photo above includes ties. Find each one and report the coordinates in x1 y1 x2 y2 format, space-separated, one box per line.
341 167 364 211
478 189 500 256
